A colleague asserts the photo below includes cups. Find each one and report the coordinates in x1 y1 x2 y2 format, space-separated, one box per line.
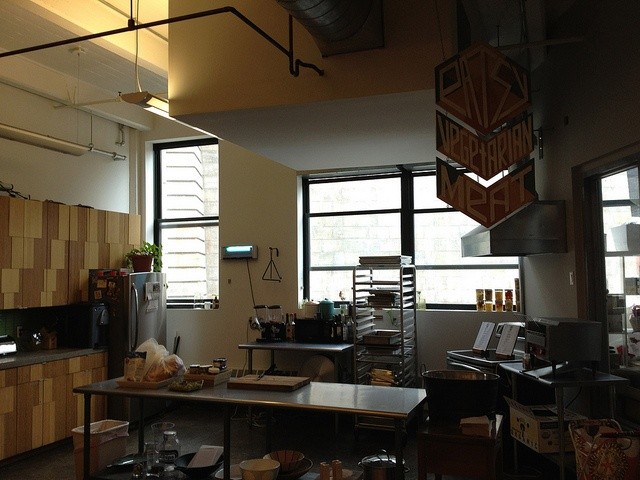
418 298 426 311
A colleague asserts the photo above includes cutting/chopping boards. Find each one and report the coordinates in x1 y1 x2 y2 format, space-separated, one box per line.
227 373 311 393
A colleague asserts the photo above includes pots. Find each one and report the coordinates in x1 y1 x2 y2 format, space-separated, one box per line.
358 449 410 480
173 451 223 477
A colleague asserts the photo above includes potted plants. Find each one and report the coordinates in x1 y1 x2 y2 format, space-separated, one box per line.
123 242 163 272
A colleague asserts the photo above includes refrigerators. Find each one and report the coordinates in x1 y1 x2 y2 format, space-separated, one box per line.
88 269 166 430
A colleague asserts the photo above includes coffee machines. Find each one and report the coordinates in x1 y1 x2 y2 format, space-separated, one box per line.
253 305 288 343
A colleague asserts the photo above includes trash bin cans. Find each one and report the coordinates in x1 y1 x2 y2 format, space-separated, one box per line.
71 419 129 480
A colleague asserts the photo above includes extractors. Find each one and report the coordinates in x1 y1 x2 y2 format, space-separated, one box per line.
461 199 568 257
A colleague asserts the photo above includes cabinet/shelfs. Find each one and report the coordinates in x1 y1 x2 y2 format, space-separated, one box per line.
0 351 108 463
0 196 141 311
607 249 640 366
354 256 420 433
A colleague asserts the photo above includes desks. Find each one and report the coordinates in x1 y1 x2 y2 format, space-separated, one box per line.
73 377 426 480
238 339 354 433
500 363 628 480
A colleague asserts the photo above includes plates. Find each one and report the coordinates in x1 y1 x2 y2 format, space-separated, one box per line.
359 255 412 265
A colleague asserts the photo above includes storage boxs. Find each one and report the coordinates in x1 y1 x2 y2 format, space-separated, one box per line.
503 395 588 454
423 371 498 426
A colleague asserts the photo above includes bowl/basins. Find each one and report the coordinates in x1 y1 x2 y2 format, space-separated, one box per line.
262 451 304 467
235 458 279 479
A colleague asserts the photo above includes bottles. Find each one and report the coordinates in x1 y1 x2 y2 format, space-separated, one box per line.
330 313 353 343
495 289 504 301
477 301 484 311
286 313 296 340
485 288 493 301
515 278 520 289
303 299 319 319
497 301 503 313
523 353 530 369
476 288 483 300
485 302 494 313
183 357 227 374
505 301 514 313
142 440 158 471
515 302 520 311
504 288 513 300
213 296 219 309
320 297 334 318
514 290 521 302
158 433 179 469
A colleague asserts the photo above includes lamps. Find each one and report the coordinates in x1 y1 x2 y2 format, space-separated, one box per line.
120 91 224 141
